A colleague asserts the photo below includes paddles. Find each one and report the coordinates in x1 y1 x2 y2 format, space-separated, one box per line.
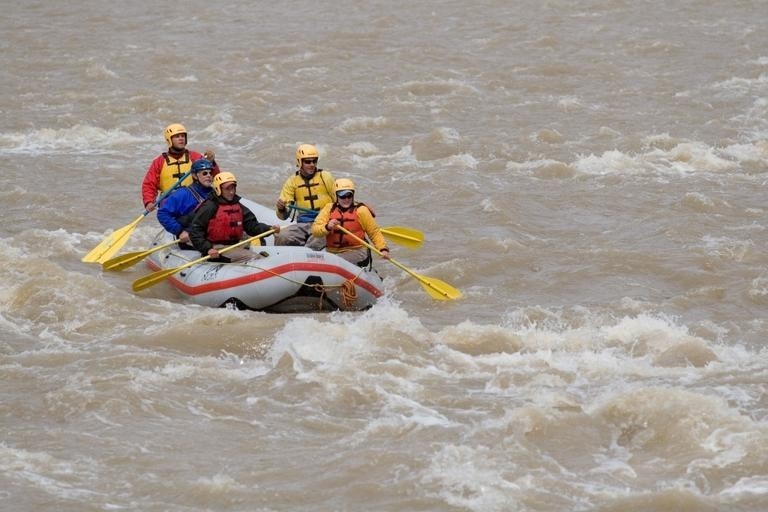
102 239 182 272
336 224 463 300
133 229 276 292
81 154 209 263
284 202 425 249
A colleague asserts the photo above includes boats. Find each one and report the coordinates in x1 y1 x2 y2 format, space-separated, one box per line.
146 196 384 313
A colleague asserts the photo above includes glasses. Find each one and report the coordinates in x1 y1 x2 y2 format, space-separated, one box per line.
339 195 352 199
202 172 213 176
303 160 317 164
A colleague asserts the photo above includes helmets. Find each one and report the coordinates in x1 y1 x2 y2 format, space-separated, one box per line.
164 123 187 147
332 178 354 202
213 171 237 196
191 159 213 174
296 146 319 170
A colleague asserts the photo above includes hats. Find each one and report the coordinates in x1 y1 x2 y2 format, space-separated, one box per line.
337 190 353 197
221 182 237 189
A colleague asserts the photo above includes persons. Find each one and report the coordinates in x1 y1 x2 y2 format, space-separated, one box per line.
311 179 391 270
141 124 220 211
159 159 217 250
275 144 336 246
191 172 279 262
